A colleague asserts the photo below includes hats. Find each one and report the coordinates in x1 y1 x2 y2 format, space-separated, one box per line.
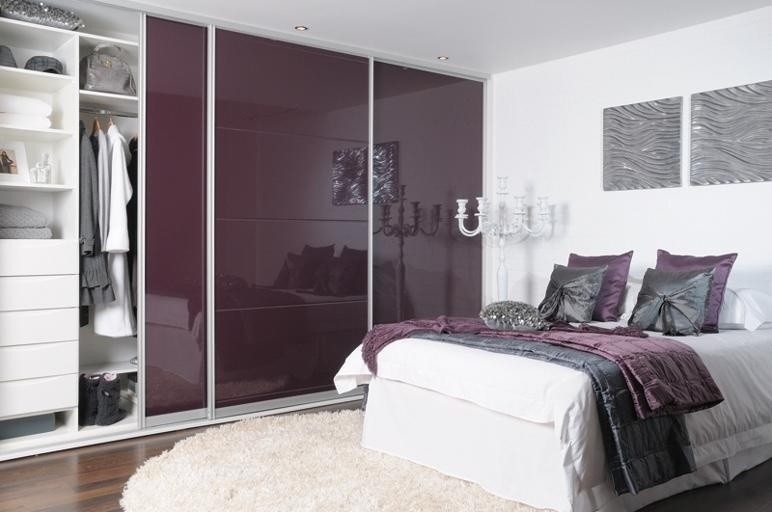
0 45 17 67
24 56 62 74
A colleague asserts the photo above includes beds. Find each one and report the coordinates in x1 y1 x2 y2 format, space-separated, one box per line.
333 276 772 510
146 260 399 387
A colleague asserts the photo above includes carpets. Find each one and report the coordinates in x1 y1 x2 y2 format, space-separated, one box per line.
119 409 558 512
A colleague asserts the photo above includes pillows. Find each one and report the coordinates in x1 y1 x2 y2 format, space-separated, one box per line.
656 248 730 327
480 301 544 332
273 243 377 297
568 250 633 321
538 264 607 323
619 282 768 333
629 268 713 338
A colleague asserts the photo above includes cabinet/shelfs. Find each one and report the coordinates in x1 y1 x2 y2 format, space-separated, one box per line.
212 27 483 417
78 4 211 434
1 2 79 424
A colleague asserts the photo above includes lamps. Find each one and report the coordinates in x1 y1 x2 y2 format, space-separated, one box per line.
374 185 441 322
454 177 550 300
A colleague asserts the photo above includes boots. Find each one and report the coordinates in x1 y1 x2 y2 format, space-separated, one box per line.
79 373 127 426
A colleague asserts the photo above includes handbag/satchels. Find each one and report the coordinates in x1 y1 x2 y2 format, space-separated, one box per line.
80 44 135 95
